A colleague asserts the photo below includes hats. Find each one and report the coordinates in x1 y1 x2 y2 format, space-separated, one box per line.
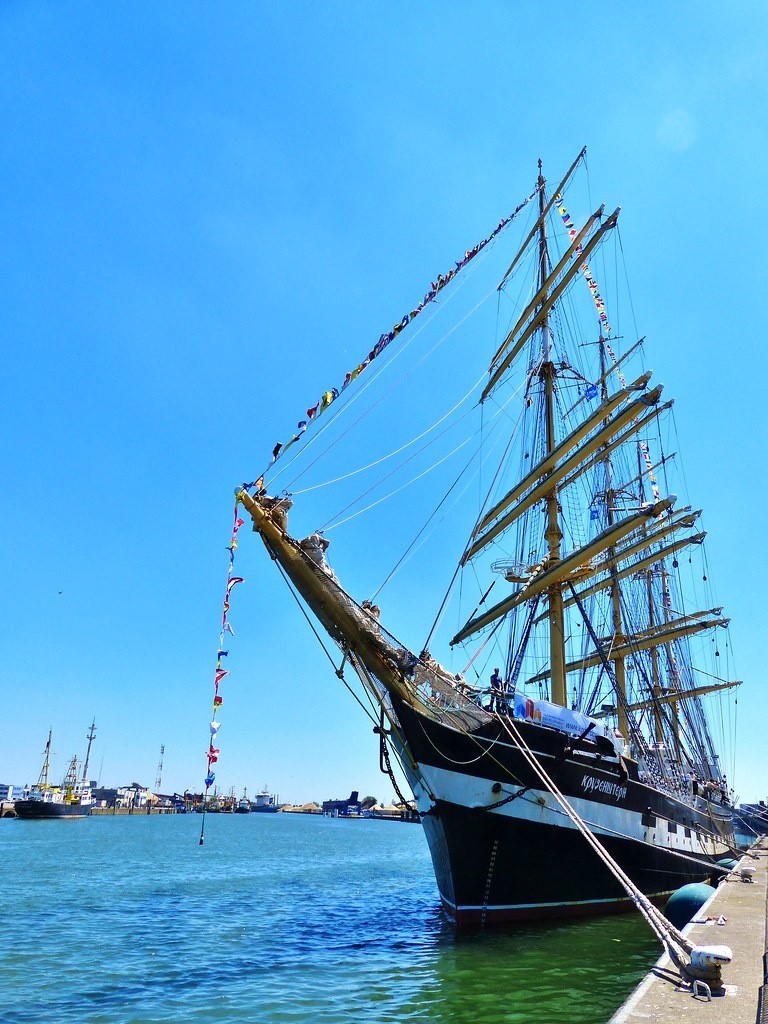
494 668 499 671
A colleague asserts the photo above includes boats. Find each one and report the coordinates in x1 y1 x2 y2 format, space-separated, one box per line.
11 754 99 819
738 802 768 831
195 787 278 815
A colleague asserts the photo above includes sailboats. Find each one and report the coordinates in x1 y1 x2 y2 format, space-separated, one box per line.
230 142 743 929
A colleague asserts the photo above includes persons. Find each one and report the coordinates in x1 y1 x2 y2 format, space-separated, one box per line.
614 728 622 739
491 668 504 713
680 770 729 806
